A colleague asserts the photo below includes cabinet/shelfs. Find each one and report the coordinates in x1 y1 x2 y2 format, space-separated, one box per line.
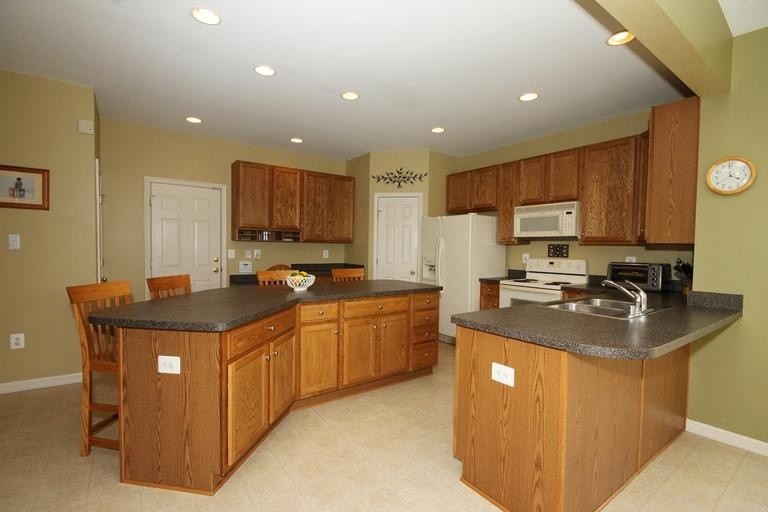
577 136 637 246
440 165 495 216
636 130 647 247
404 282 443 380
510 146 581 205
297 167 354 246
230 158 302 241
477 277 500 313
645 95 702 246
337 280 411 398
495 160 531 245
289 281 345 410
114 287 301 495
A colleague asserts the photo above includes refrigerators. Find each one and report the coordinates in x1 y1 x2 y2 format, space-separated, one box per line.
417 212 510 346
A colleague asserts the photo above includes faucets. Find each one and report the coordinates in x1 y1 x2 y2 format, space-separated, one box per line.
602 278 641 312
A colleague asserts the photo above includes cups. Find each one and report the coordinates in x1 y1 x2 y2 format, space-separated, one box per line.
625 256 636 264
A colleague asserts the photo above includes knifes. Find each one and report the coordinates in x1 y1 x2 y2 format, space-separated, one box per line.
673 257 692 283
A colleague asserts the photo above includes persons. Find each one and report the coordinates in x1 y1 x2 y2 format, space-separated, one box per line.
14 177 22 189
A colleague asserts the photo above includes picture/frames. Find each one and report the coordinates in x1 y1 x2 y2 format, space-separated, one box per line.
1 162 50 211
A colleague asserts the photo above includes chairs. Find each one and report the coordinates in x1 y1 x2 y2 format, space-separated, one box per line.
256 269 297 285
329 264 363 282
65 277 137 458
145 272 191 301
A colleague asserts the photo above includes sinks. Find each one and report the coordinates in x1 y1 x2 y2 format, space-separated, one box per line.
577 298 635 311
547 302 627 319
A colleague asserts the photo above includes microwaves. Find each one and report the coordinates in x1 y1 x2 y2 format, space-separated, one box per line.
513 202 581 241
606 261 671 293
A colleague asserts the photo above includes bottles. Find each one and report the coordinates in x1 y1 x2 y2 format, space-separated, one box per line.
259 231 272 240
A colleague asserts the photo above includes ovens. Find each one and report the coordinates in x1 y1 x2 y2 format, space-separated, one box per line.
498 286 564 309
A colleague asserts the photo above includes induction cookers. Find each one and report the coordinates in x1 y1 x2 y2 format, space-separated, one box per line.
500 277 575 291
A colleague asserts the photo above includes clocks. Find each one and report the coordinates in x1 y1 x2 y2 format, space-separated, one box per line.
703 156 755 197
